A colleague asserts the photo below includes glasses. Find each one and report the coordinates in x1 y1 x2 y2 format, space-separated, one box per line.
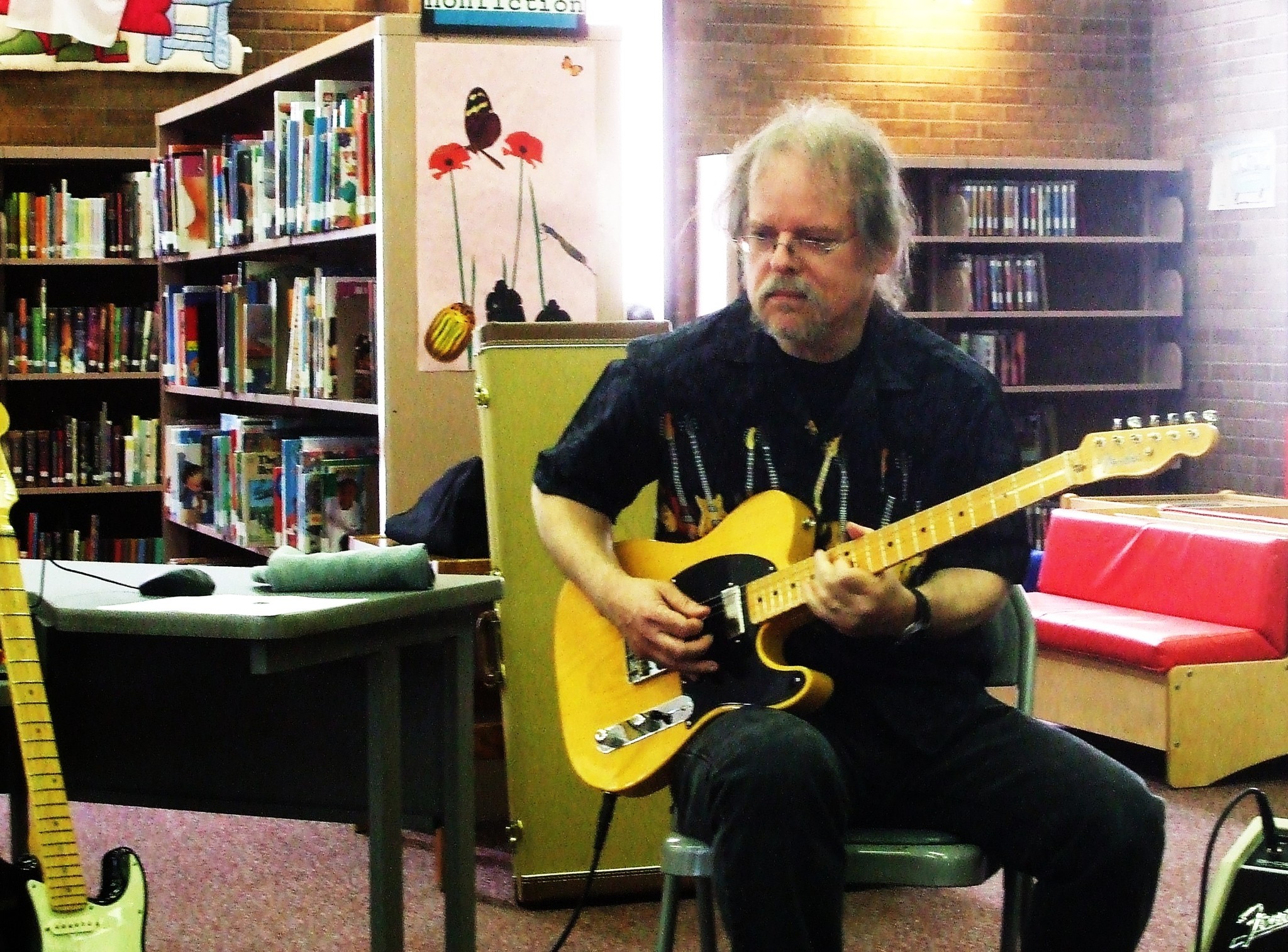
732 216 866 256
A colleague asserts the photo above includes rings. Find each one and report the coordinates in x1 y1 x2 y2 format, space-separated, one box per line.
831 603 841 614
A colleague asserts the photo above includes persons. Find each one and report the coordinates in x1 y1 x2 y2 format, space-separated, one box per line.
531 108 1168 952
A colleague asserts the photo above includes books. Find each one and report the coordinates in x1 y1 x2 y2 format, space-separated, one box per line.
160 261 377 400
1023 504 1050 551
0 277 159 372
952 250 1048 311
20 511 165 564
164 415 379 552
951 180 1076 235
0 170 155 258
1013 403 1058 467
150 79 374 252
0 401 159 487
973 330 1025 386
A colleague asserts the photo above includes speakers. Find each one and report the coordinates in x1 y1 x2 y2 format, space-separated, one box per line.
1193 813 1288 952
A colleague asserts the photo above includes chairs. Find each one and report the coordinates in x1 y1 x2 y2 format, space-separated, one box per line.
656 580 1039 952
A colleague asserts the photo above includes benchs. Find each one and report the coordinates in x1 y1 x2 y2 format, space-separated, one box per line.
986 510 1288 787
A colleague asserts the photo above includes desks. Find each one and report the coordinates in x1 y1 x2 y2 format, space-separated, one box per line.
1 560 506 952
347 533 502 890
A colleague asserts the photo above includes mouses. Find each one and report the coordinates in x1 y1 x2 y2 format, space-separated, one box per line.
139 568 215 597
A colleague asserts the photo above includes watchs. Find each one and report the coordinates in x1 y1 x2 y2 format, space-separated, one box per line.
895 589 929 647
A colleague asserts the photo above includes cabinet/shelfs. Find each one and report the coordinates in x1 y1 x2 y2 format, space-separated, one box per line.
694 155 1187 392
0 17 389 569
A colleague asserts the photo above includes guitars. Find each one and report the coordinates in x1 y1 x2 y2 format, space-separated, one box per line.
0 401 151 952
552 410 1224 798
